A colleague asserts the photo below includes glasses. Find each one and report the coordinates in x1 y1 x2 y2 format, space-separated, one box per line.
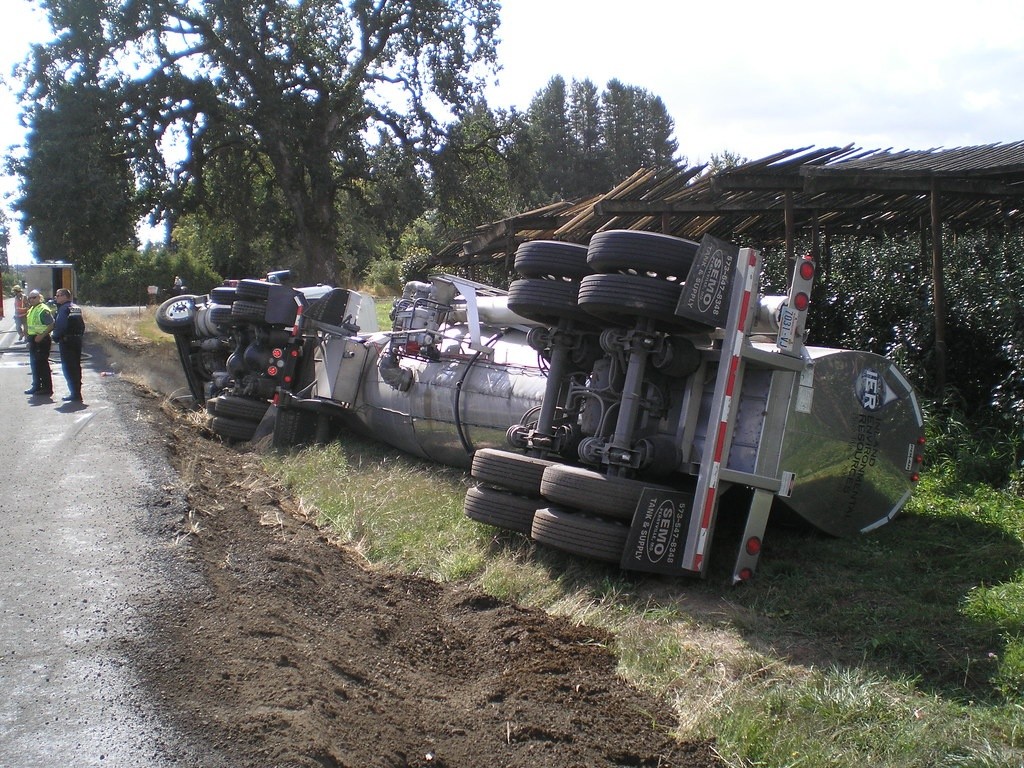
29 296 36 299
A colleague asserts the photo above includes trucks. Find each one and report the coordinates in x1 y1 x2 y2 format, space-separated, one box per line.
26 263 78 304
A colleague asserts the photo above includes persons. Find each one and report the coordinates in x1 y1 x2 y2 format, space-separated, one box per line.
52 289 86 401
46 299 57 319
24 289 55 395
173 276 182 291
11 284 32 343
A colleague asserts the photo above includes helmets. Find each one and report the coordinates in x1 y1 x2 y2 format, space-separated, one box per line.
13 285 21 292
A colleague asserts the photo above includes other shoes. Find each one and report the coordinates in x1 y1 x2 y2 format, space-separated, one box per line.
18 332 25 340
62 396 82 401
35 389 52 396
64 401 82 406
24 386 42 394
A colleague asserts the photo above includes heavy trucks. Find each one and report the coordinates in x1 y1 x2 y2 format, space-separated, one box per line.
155 227 927 587
